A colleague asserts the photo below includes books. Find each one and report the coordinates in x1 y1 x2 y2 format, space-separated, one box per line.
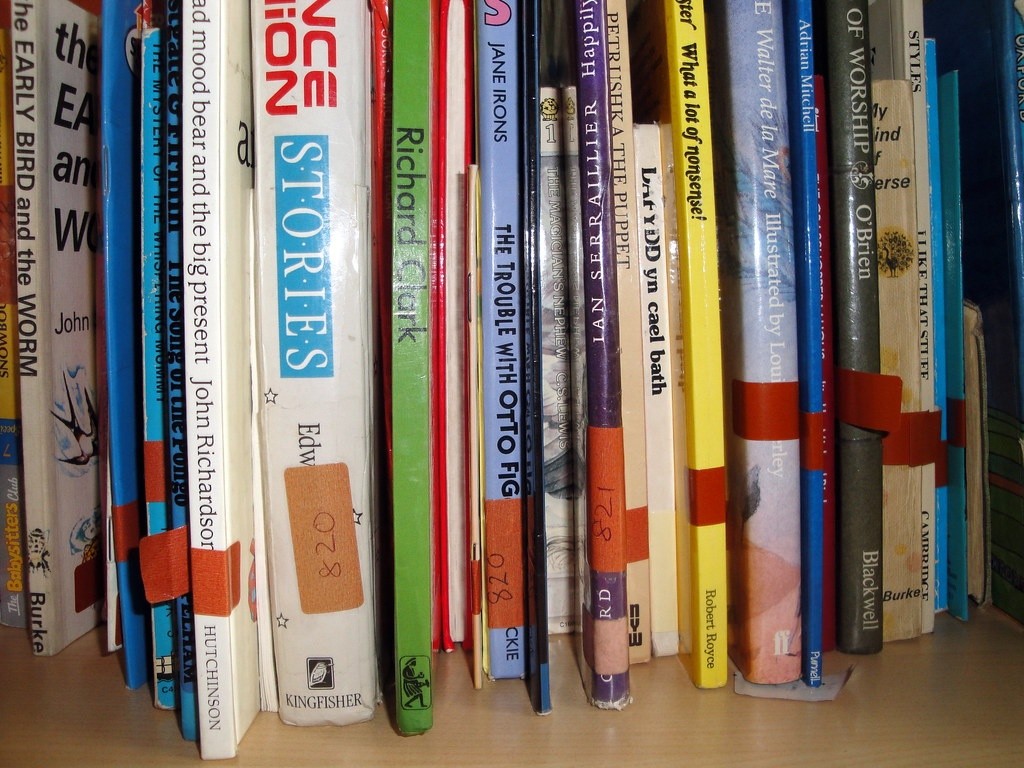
2 0 1023 768
